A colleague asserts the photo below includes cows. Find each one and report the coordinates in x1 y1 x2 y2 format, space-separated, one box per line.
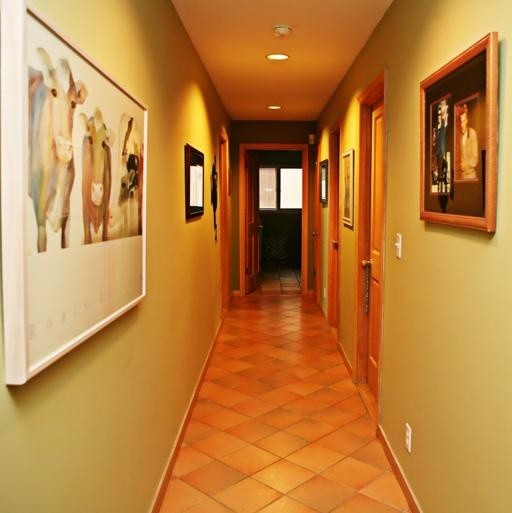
76 101 115 246
28 44 88 254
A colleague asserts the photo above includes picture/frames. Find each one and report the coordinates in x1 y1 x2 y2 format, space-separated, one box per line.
186 144 205 218
319 149 356 227
419 32 498 234
1 0 146 386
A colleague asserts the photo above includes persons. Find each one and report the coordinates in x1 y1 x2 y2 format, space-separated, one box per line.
431 105 450 192
460 101 481 180
